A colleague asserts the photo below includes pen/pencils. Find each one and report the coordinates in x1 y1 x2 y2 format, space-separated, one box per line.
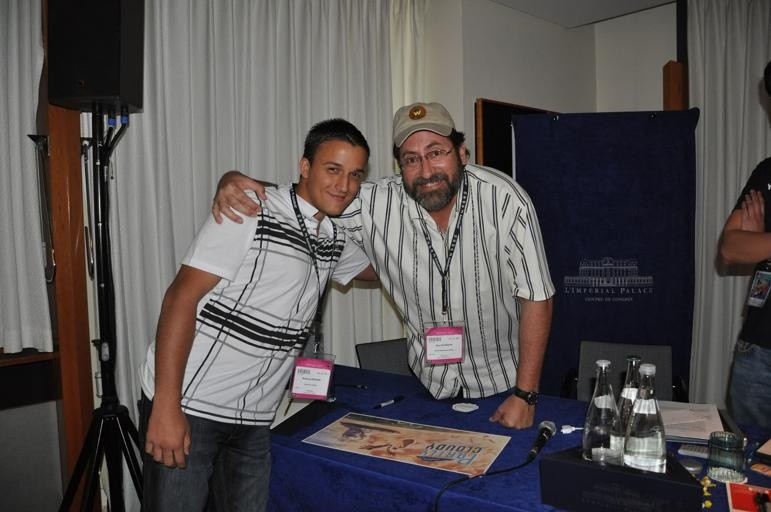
373 395 405 409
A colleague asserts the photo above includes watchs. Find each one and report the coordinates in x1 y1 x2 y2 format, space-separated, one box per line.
514 388 539 405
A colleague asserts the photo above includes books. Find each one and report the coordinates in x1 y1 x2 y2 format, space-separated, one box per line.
658 400 725 445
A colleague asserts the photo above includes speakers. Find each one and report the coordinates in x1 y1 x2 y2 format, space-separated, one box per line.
44 0 146 112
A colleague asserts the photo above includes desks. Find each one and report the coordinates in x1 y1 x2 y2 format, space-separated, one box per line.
267 362 771 512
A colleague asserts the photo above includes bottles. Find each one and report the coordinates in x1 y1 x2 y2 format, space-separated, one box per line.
581 359 626 468
616 355 642 434
624 363 667 473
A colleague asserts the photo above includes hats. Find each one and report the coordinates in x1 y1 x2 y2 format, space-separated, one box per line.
392 102 456 147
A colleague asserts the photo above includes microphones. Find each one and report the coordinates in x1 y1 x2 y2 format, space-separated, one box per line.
525 418 558 461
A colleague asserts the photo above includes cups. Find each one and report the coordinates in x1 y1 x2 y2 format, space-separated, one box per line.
705 430 747 485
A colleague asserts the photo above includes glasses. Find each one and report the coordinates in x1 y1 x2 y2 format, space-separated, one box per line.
396 145 458 171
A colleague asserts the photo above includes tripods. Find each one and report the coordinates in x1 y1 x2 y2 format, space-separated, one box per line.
60 112 145 511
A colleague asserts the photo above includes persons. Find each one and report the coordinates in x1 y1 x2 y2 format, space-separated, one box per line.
210 102 556 432
718 58 771 437
139 117 382 512
